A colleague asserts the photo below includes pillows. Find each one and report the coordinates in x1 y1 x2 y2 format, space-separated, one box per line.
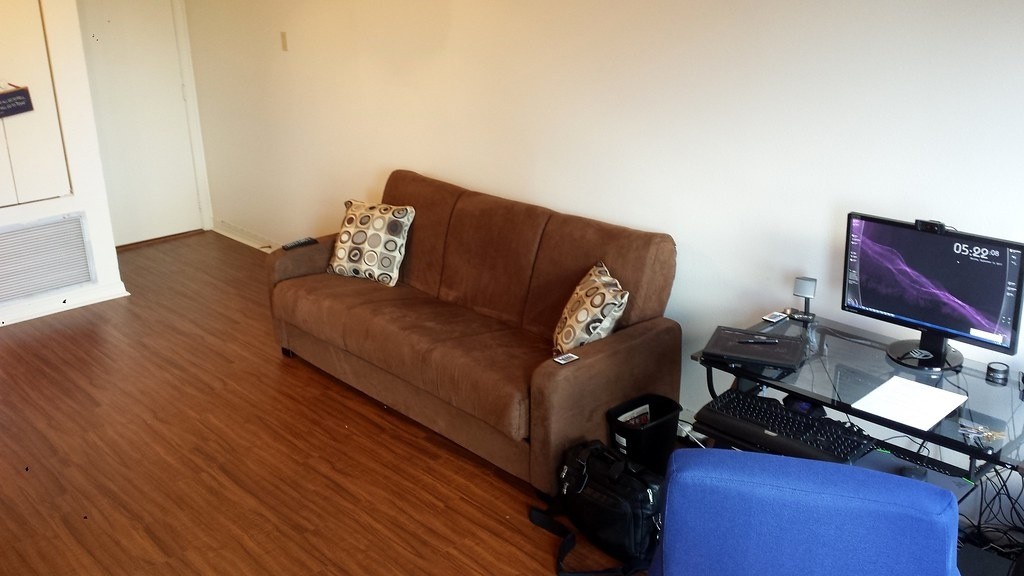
325 199 415 286
552 262 629 359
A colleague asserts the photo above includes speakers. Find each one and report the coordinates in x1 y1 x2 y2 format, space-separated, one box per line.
789 277 818 322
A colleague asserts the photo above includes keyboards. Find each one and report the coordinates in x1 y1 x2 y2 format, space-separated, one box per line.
694 388 878 467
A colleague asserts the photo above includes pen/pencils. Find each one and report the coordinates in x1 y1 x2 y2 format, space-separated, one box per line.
737 339 779 343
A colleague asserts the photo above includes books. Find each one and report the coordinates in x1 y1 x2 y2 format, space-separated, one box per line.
702 323 806 371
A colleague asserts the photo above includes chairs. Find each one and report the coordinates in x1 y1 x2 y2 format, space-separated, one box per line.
653 447 962 576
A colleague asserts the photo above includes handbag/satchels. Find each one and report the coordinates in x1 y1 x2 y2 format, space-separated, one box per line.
529 441 667 576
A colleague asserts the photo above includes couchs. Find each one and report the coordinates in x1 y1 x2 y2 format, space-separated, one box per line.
264 168 683 489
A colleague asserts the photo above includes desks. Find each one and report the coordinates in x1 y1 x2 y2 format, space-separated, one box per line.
691 306 1024 576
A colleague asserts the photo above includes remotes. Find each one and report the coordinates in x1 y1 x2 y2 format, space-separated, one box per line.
283 238 319 250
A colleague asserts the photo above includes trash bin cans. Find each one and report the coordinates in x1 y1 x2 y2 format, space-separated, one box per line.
606 391 684 459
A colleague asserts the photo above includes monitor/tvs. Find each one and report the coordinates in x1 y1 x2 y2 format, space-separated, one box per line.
841 213 1024 370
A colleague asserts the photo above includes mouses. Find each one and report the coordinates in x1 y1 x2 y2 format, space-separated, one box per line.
899 465 928 483
